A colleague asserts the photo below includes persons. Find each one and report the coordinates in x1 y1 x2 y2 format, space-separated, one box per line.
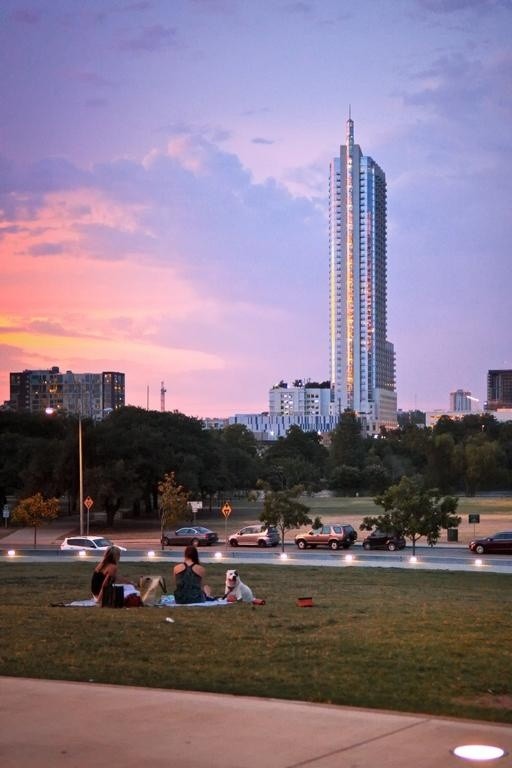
91 546 140 604
172 545 212 603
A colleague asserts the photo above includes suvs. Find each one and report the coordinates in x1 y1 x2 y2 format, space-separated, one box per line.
295 524 357 550
60 536 127 552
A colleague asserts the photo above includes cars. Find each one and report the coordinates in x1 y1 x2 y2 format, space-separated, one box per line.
362 526 406 551
228 524 281 548
469 531 512 555
161 526 219 547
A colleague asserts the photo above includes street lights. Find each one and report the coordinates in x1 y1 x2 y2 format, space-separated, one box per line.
45 407 114 536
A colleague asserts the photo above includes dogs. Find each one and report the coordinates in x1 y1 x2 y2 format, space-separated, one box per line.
224 569 255 604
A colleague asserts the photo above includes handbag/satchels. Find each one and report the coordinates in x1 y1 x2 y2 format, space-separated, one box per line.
140 574 166 606
100 582 125 610
